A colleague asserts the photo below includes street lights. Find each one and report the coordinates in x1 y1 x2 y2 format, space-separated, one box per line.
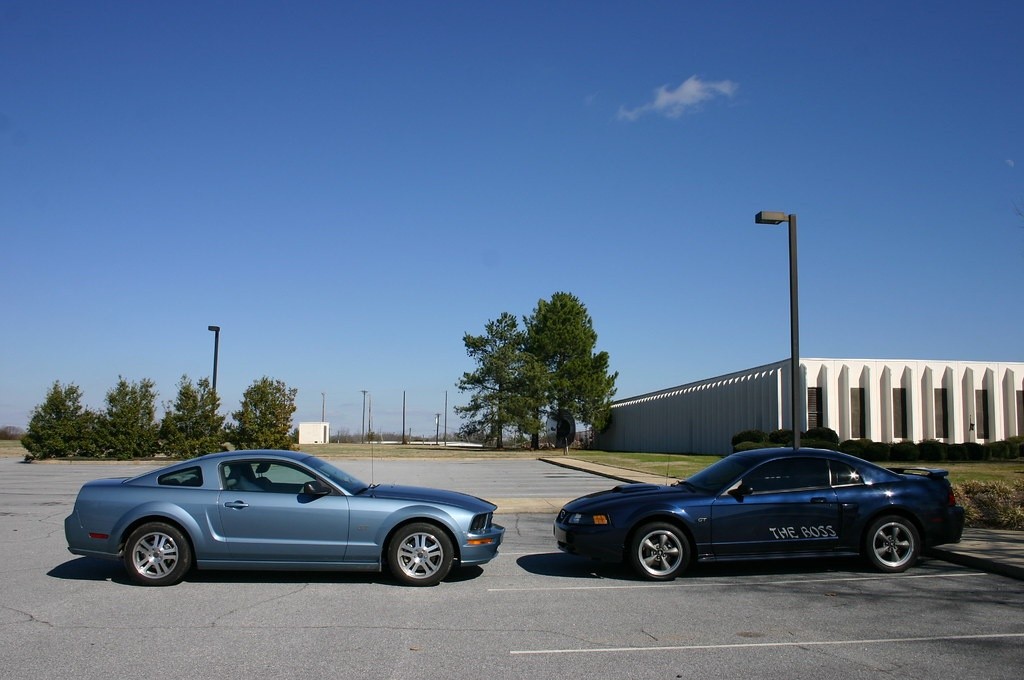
322 392 325 422
756 211 801 448
208 325 220 418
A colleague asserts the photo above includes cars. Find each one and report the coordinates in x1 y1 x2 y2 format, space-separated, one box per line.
553 446 967 580
64 450 505 586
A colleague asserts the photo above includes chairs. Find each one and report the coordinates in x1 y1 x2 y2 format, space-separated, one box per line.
162 462 276 492
